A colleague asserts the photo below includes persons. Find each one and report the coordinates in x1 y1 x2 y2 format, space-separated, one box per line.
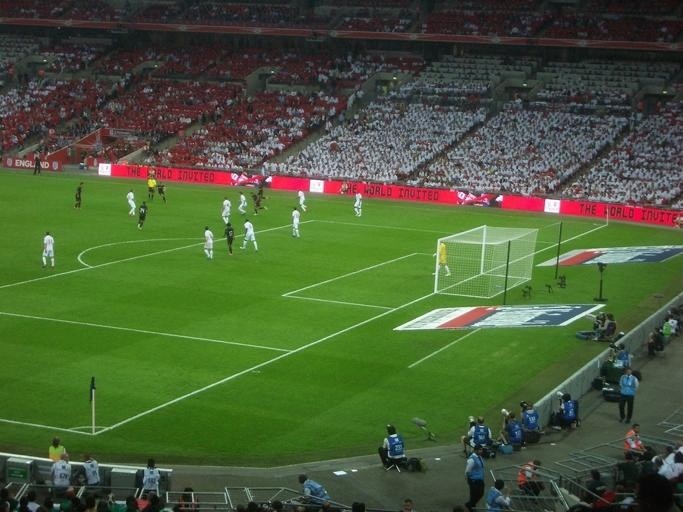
433 242 452 279
1 1 267 171
245 501 283 512
1 437 200 512
618 367 640 425
263 1 683 218
517 459 541 497
240 219 261 252
202 225 214 259
378 424 406 470
34 154 41 175
467 393 576 449
224 223 239 255
40 233 57 271
222 181 268 223
298 475 331 506
592 313 616 341
75 182 84 209
461 444 511 511
647 309 680 358
584 423 682 510
400 500 414 508
290 207 300 238
126 172 167 230
617 344 629 368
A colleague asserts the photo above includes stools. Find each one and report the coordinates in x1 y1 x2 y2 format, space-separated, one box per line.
386 458 403 472
